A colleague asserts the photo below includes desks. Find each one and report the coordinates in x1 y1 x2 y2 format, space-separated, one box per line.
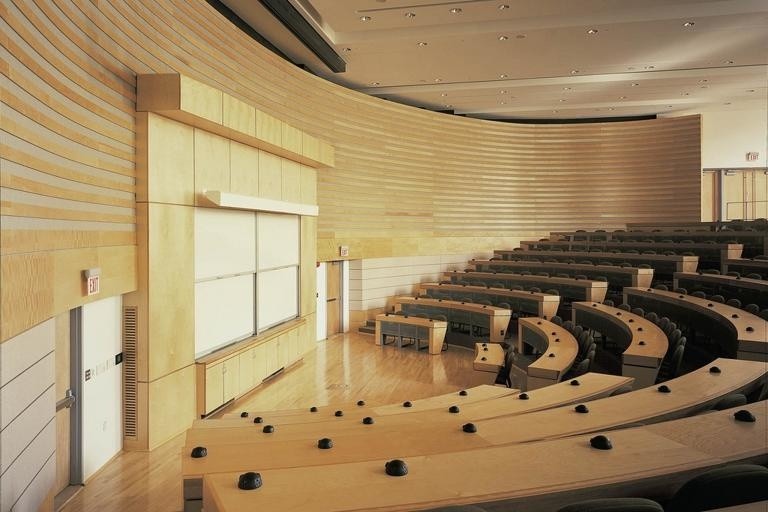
181 221 767 512
